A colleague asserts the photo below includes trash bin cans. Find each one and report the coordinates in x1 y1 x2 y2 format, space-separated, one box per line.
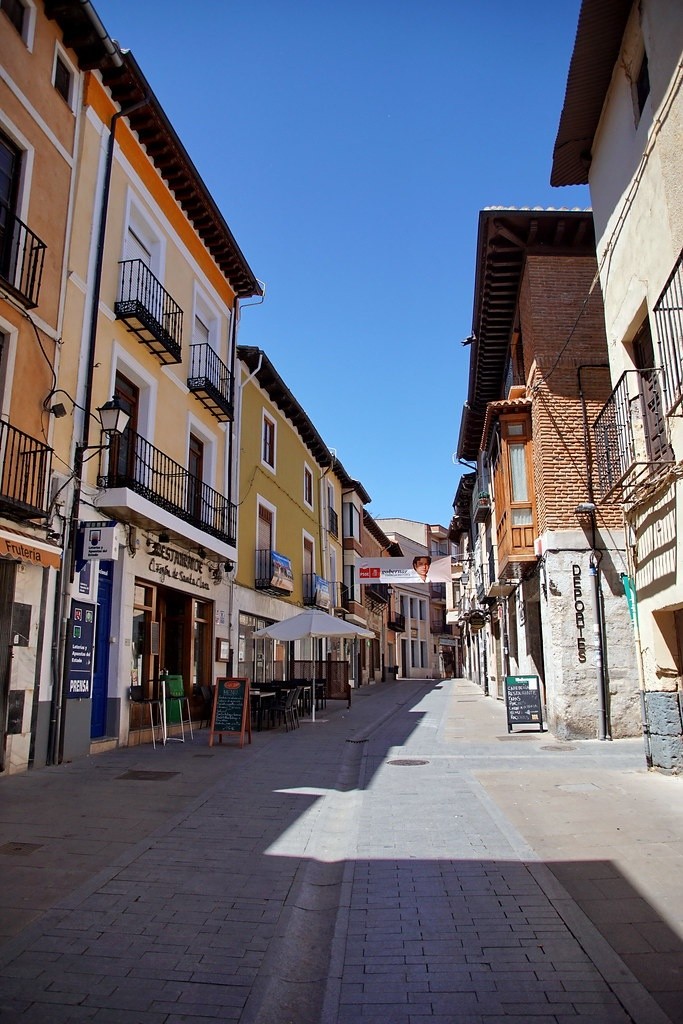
159 674 185 724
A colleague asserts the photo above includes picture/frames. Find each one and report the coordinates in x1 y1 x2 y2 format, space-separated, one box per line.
216 637 230 663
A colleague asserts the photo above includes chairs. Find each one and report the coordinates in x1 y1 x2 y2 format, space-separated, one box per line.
199 685 215 729
247 678 326 732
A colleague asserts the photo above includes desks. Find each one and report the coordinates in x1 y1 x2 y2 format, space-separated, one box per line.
315 683 324 710
249 692 277 732
281 686 310 724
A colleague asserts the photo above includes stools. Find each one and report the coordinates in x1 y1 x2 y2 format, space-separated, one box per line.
126 685 166 750
159 696 194 743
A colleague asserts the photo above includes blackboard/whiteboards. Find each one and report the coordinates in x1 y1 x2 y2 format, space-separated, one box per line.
210 677 251 735
504 675 543 723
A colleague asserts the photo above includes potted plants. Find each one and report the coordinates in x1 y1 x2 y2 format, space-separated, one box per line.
478 491 490 507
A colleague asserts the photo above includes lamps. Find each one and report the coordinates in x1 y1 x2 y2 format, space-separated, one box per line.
146 528 170 543
219 559 234 573
460 570 471 589
190 546 207 560
74 395 131 450
